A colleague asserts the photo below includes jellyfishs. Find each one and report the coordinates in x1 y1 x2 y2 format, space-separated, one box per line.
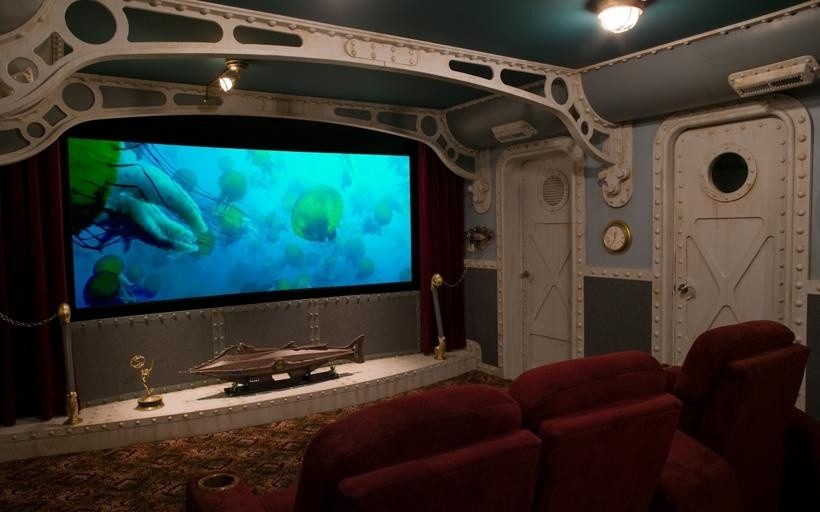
64 139 414 309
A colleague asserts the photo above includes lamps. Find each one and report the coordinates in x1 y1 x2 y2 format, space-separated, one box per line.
218 60 249 92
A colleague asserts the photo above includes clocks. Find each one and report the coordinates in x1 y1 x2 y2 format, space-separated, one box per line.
601 220 632 255
